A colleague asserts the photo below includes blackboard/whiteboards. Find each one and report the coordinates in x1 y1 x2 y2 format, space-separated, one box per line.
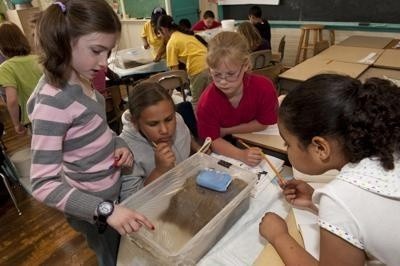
218 0 400 33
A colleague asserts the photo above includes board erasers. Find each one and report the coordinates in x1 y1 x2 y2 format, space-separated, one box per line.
358 24 369 26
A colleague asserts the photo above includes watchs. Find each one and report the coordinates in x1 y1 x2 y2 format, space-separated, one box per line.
96 199 114 235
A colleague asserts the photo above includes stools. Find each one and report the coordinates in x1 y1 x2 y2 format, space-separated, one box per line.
295 24 325 65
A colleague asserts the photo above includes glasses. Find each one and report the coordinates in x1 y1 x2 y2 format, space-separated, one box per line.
213 60 246 82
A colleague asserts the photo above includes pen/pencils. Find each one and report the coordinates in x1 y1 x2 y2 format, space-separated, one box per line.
237 138 253 149
149 140 176 166
262 152 286 186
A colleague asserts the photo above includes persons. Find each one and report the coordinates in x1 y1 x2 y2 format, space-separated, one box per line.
259 73 400 265
195 31 279 166
237 5 272 53
141 7 221 117
117 81 211 206
0 21 48 137
25 0 154 266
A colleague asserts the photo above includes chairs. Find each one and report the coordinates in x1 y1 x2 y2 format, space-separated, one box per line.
330 31 337 45
